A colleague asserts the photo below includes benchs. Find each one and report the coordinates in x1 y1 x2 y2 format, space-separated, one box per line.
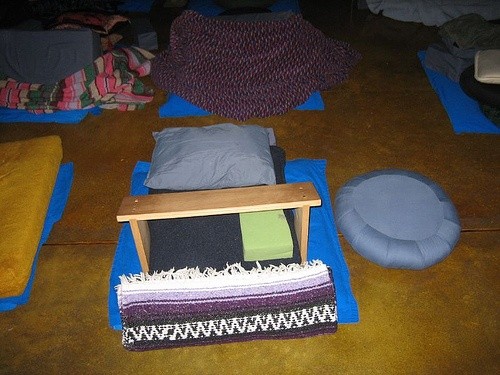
116 182 322 273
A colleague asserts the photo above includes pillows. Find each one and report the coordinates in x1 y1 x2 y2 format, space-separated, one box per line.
335 169 460 270
143 123 276 190
0 28 101 83
0 136 63 298
474 49 500 83
